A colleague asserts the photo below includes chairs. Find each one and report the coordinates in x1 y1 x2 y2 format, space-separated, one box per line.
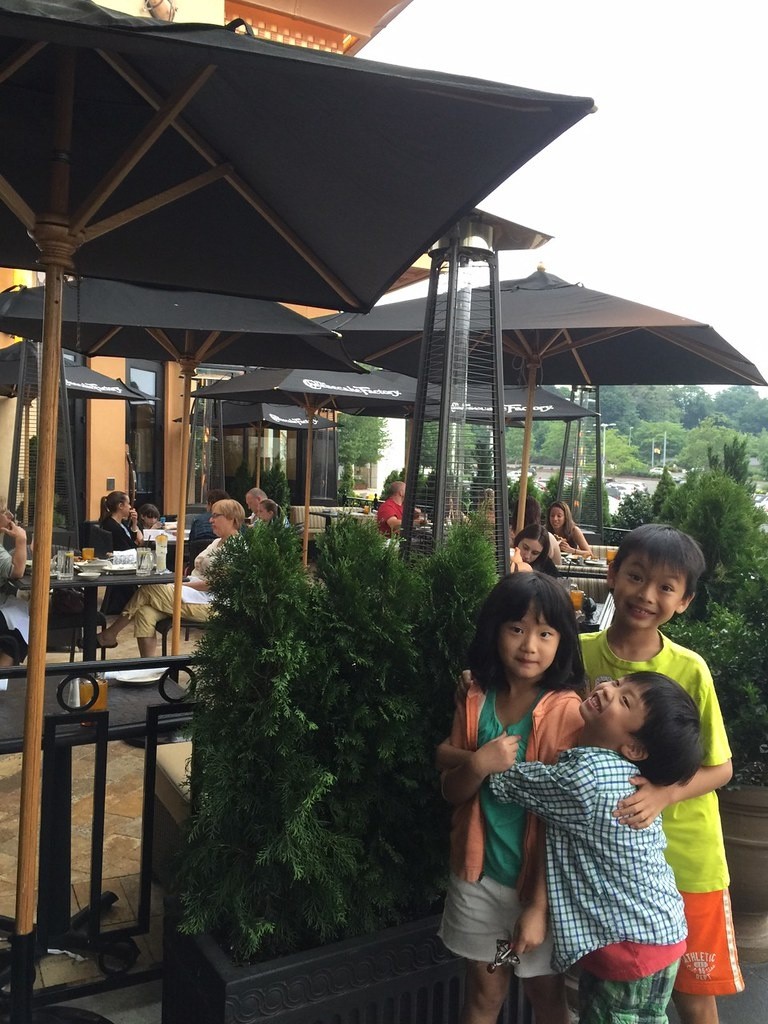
91 525 138 613
152 616 210 657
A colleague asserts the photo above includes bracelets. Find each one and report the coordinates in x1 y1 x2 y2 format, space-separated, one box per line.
130 526 139 532
574 548 577 554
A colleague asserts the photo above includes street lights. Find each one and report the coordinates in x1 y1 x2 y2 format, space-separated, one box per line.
629 427 633 445
593 423 616 483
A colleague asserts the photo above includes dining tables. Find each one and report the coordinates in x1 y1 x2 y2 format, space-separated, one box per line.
0 521 196 989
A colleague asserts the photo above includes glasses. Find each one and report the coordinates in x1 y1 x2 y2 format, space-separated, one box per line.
211 512 227 518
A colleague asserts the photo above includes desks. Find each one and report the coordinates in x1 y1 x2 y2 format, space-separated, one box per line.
309 511 381 532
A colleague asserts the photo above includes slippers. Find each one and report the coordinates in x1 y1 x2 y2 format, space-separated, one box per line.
78 635 118 647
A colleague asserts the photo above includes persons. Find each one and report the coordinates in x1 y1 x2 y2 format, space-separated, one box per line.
433 669 703 1024
99 492 145 614
247 498 283 530
136 504 165 530
0 494 30 668
508 524 560 577
184 488 247 577
437 571 589 1024
244 488 291 526
376 480 427 542
544 500 593 557
577 522 746 1024
507 495 562 565
75 498 248 658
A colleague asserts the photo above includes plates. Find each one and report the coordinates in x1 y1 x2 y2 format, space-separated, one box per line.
103 565 135 574
421 527 432 531
585 560 606 564
115 668 162 682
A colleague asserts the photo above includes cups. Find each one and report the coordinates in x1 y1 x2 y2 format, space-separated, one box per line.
136 548 157 575
156 537 167 573
607 549 618 561
364 505 369 514
571 590 584 611
52 550 74 580
79 680 108 711
81 548 94 560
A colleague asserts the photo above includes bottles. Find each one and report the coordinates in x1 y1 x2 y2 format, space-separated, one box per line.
365 496 368 506
374 494 377 509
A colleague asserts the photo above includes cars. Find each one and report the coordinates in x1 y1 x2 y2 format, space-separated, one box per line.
533 476 647 516
650 467 670 474
507 467 535 483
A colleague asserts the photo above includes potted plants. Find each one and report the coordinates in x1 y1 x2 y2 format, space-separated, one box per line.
653 527 768 916
159 453 540 1024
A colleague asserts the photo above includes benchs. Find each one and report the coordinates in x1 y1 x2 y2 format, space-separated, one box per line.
289 506 363 544
554 564 612 604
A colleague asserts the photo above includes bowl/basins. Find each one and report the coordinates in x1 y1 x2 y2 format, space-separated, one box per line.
79 564 108 572
78 572 101 580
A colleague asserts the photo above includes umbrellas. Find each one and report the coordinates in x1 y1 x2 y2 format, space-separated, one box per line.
0 4 768 1024
